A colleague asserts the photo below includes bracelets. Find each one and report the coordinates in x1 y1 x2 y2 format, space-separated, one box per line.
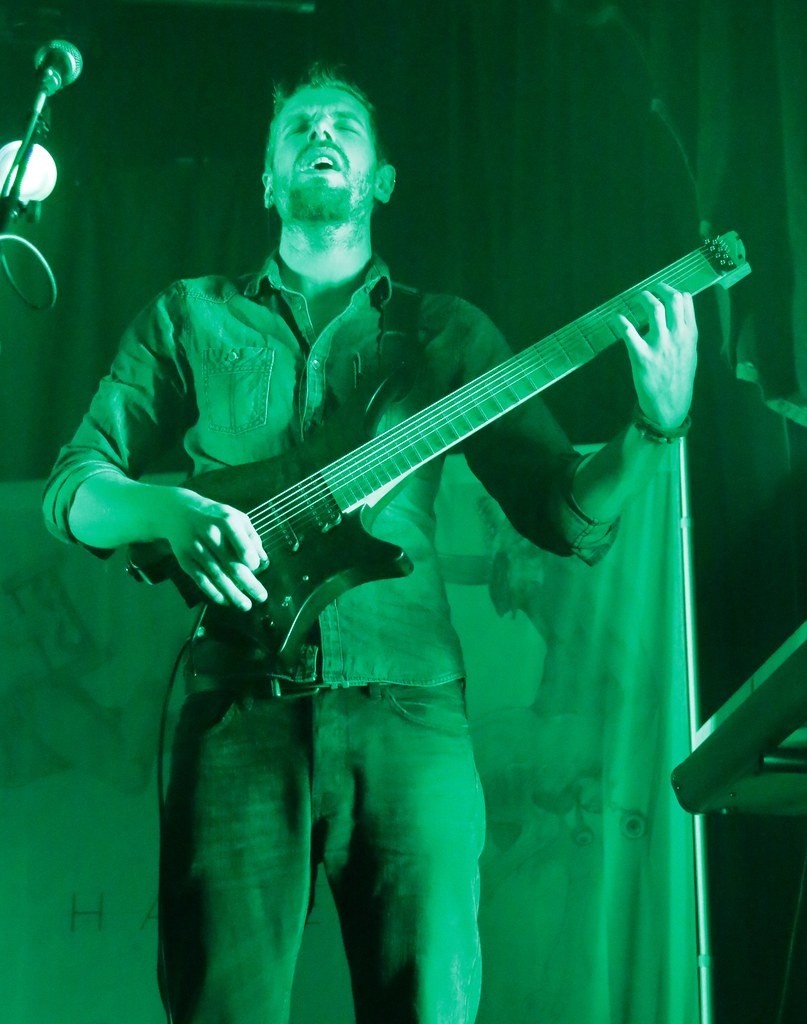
631 400 691 445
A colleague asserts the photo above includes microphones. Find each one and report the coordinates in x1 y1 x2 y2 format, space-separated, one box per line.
35 39 83 97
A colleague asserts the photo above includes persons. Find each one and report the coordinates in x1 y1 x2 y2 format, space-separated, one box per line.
41 62 699 1024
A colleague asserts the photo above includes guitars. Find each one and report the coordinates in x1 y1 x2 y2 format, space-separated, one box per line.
127 227 750 698
